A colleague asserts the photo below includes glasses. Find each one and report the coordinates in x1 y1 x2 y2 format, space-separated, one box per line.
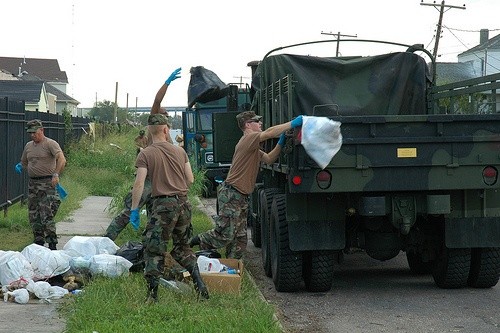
248 119 260 123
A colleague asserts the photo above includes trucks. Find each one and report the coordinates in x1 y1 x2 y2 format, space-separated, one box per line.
238 39 500 292
182 87 253 198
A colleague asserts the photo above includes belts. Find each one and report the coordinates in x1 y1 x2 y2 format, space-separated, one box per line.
29 176 52 180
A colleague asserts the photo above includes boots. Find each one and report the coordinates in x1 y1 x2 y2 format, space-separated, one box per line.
192 270 210 299
145 278 160 302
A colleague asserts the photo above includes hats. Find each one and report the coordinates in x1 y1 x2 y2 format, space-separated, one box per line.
139 129 145 136
25 119 42 133
147 114 172 127
236 111 262 127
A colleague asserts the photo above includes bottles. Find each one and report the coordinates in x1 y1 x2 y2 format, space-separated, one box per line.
222 268 240 274
197 255 227 273
72 290 85 295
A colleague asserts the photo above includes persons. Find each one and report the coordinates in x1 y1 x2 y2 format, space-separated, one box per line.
188 111 302 261
16 119 66 251
134 130 148 158
130 114 211 303
103 67 181 246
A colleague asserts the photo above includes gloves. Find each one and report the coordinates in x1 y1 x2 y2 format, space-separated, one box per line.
56 183 67 200
15 162 23 175
163 67 182 86
278 131 287 147
130 207 142 231
290 115 306 128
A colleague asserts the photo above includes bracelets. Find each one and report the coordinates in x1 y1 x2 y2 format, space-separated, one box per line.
52 174 58 177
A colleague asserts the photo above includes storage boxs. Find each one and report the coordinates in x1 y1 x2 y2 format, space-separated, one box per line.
184 259 243 294
